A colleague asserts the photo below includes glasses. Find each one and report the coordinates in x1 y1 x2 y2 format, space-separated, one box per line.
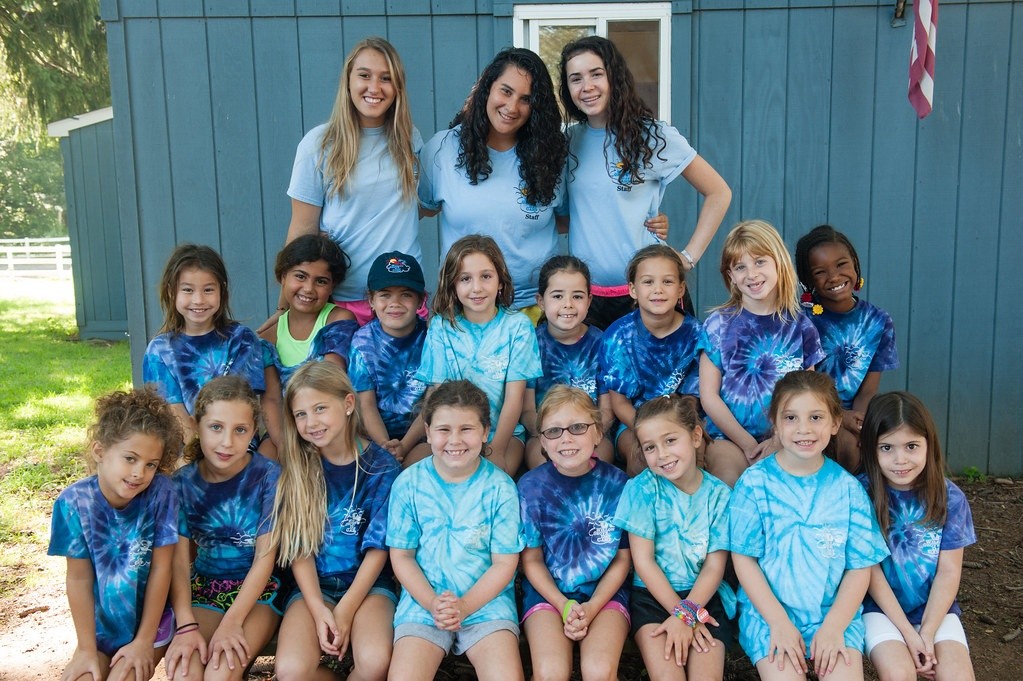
540 422 596 439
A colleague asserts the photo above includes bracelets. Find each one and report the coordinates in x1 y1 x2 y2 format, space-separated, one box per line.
681 250 695 269
563 600 575 622
176 623 200 635
672 599 709 628
277 307 285 310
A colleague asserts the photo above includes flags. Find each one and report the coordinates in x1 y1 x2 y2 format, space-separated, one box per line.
907 0 938 119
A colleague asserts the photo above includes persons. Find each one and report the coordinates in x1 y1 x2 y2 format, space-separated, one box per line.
142 245 266 475
554 35 732 332
165 376 284 681
418 47 568 328
519 255 615 471
516 384 633 681
728 370 891 681
347 250 435 469
854 391 975 681
607 393 737 681
257 235 360 461
258 360 403 681
794 225 902 471
255 38 429 334
47 388 182 681
694 220 826 490
412 234 543 468
385 379 527 681
598 244 706 478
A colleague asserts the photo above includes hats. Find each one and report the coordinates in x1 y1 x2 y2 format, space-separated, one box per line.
366 251 424 299
